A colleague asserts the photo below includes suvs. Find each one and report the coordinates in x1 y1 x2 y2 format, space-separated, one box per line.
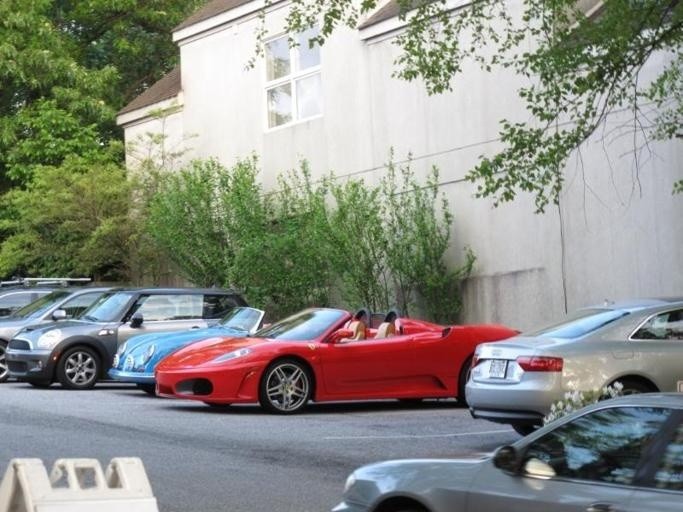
0 277 250 388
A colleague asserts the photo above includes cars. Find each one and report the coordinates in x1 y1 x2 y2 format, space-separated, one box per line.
463 296 683 435
332 392 683 512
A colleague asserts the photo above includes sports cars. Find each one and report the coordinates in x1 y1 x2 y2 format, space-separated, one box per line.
108 306 281 395
150 305 524 412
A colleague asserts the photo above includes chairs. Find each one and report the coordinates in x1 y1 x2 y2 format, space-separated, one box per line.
373 321 396 339
339 322 366 344
